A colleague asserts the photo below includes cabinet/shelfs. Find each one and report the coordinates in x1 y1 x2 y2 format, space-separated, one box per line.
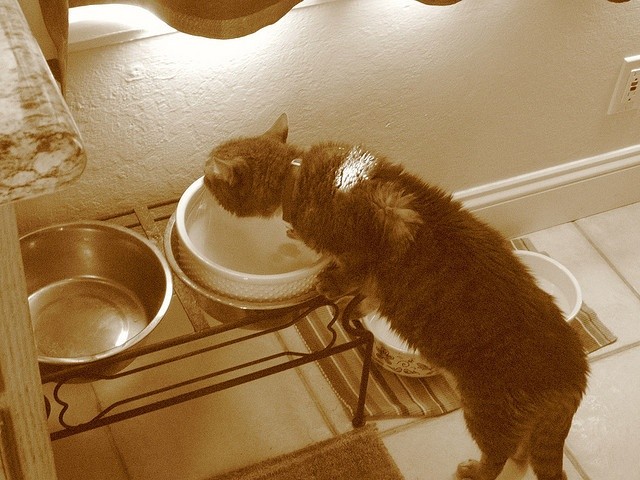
43 194 373 442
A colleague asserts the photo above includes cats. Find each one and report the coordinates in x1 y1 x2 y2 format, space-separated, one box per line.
203 113 590 480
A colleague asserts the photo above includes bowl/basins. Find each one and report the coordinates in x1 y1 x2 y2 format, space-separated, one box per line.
19 221 174 385
173 174 334 302
358 310 441 379
163 207 321 331
510 249 584 323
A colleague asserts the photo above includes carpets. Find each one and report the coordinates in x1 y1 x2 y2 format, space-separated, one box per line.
202 424 405 479
296 236 617 418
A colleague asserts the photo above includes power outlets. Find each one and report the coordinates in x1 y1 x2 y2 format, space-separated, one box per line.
606 55 640 115
